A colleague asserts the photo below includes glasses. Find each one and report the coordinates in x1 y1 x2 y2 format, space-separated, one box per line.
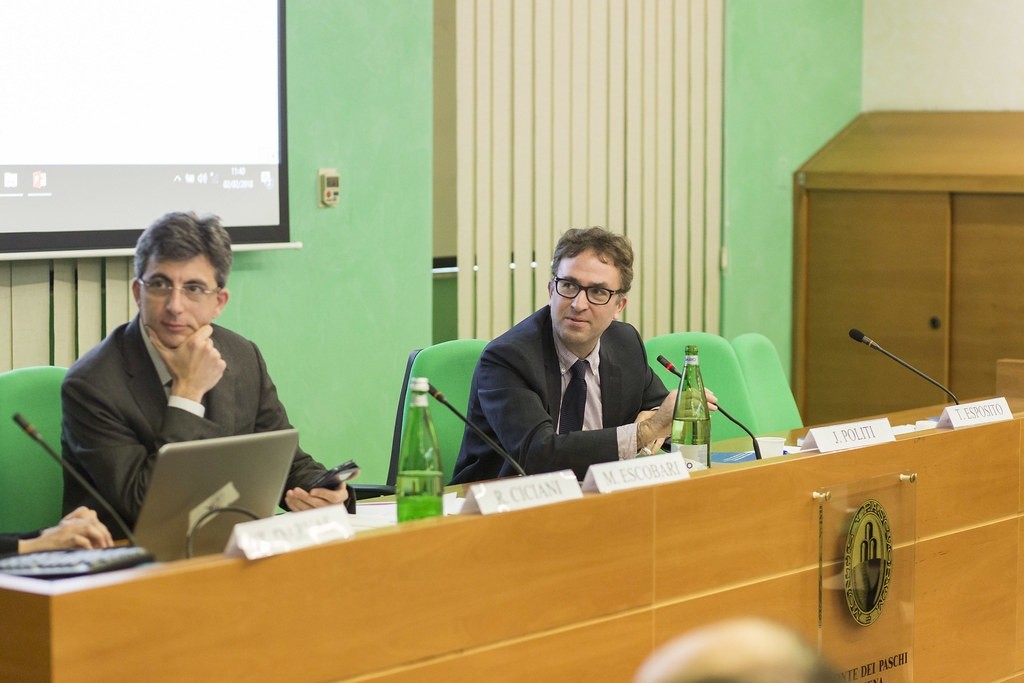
555 270 625 306
137 277 223 301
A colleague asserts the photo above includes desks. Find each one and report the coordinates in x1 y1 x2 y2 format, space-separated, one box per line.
1 396 1024 682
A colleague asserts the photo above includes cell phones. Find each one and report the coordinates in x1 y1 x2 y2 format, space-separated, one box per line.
307 460 358 492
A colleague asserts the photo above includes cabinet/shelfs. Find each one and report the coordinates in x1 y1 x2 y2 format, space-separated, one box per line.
791 108 1023 427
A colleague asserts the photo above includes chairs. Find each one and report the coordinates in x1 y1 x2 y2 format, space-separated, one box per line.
642 332 759 449
731 333 804 435
397 339 492 487
1 365 69 536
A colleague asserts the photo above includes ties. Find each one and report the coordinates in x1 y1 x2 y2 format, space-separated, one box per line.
559 359 590 436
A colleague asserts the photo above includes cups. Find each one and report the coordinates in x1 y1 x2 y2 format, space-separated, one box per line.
754 437 786 459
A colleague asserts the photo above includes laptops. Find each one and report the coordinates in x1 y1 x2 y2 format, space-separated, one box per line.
135 428 300 562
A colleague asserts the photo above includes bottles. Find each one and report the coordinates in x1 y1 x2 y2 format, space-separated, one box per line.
671 345 711 472
396 378 444 523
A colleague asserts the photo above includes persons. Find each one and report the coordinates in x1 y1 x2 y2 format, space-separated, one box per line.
62 211 361 541
445 226 719 487
0 505 117 558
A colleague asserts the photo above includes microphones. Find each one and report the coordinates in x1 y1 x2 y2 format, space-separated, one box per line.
849 329 959 405
428 383 527 477
12 414 138 547
657 354 762 460
